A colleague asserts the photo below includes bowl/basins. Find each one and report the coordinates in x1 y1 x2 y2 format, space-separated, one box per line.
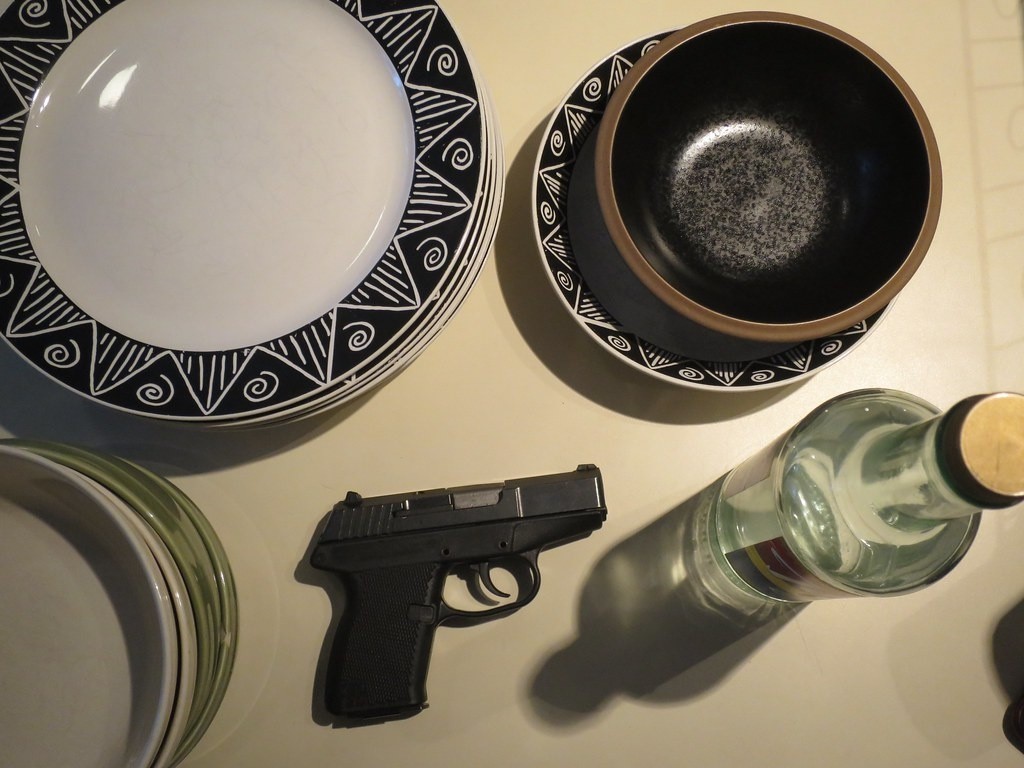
596 11 942 339
2 437 240 768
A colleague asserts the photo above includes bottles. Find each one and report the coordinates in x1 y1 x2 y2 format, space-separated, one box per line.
678 387 1024 634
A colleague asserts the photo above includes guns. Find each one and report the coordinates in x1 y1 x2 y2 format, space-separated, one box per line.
308 463 608 723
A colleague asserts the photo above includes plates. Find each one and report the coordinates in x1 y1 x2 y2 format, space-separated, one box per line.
533 27 896 394
0 0 505 428
